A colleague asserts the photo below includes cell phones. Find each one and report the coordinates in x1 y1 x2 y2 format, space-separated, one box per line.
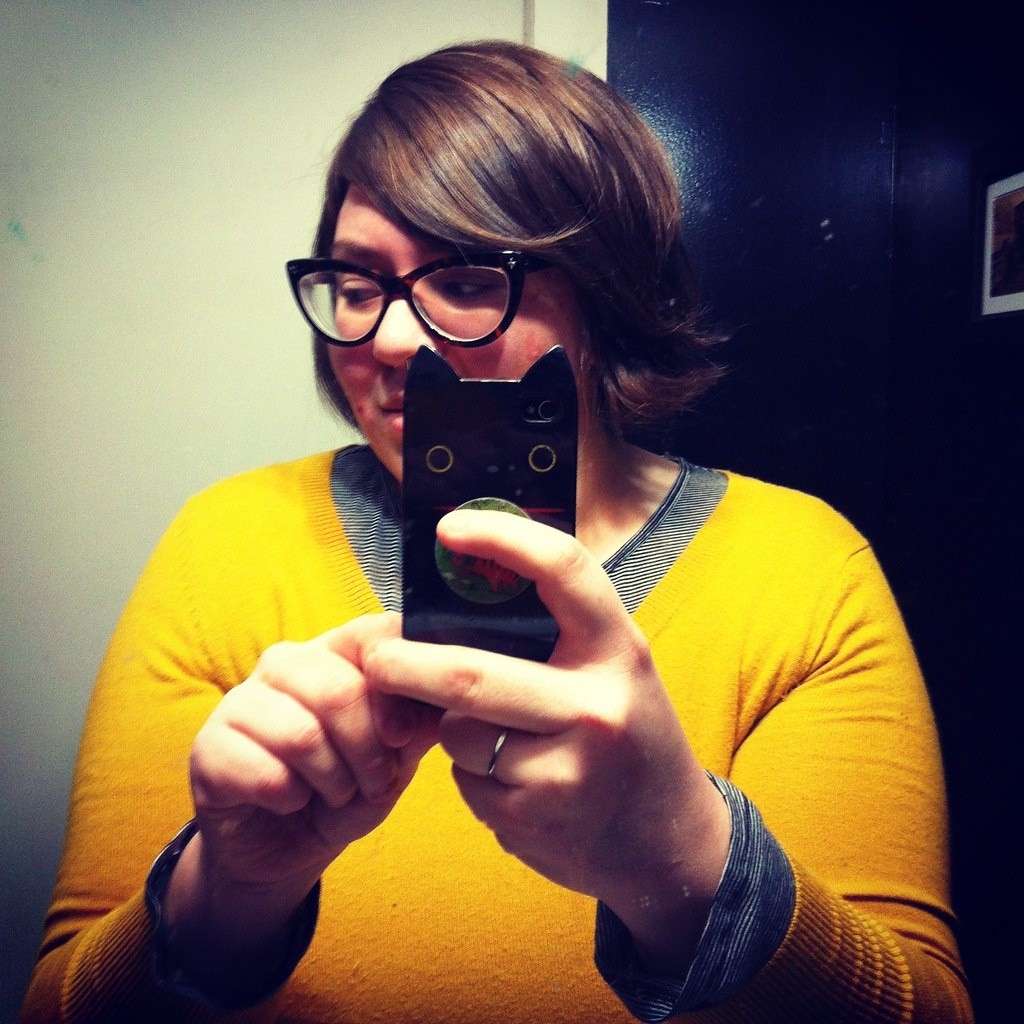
401 344 578 665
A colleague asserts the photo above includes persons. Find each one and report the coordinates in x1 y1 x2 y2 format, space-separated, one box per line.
23 42 976 1024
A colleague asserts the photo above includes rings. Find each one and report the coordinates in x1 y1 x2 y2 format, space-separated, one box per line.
487 730 505 777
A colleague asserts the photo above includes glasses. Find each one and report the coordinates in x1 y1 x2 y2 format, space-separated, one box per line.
286 249 556 347
